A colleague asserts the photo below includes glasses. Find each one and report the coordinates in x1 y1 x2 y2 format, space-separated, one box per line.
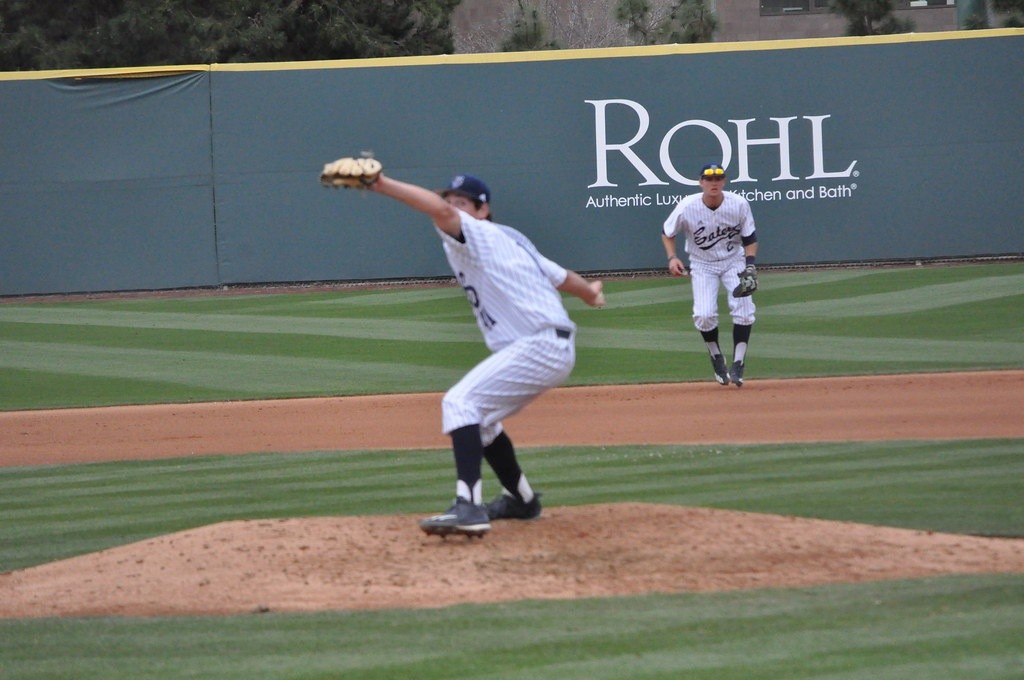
701 168 725 177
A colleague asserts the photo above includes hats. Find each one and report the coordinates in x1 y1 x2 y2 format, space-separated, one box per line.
442 174 490 203
700 161 726 178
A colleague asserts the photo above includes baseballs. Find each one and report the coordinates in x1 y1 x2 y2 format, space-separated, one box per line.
597 292 604 301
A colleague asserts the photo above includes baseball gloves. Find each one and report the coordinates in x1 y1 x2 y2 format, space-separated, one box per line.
732 269 758 298
319 159 382 190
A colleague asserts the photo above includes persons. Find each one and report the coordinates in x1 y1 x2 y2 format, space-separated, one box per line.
318 155 607 547
661 163 760 389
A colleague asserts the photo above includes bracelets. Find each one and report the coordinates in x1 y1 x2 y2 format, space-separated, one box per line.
668 255 676 262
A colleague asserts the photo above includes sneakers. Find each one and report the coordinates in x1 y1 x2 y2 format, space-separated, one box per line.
710 354 730 385
729 360 744 387
419 497 491 537
485 492 543 519
